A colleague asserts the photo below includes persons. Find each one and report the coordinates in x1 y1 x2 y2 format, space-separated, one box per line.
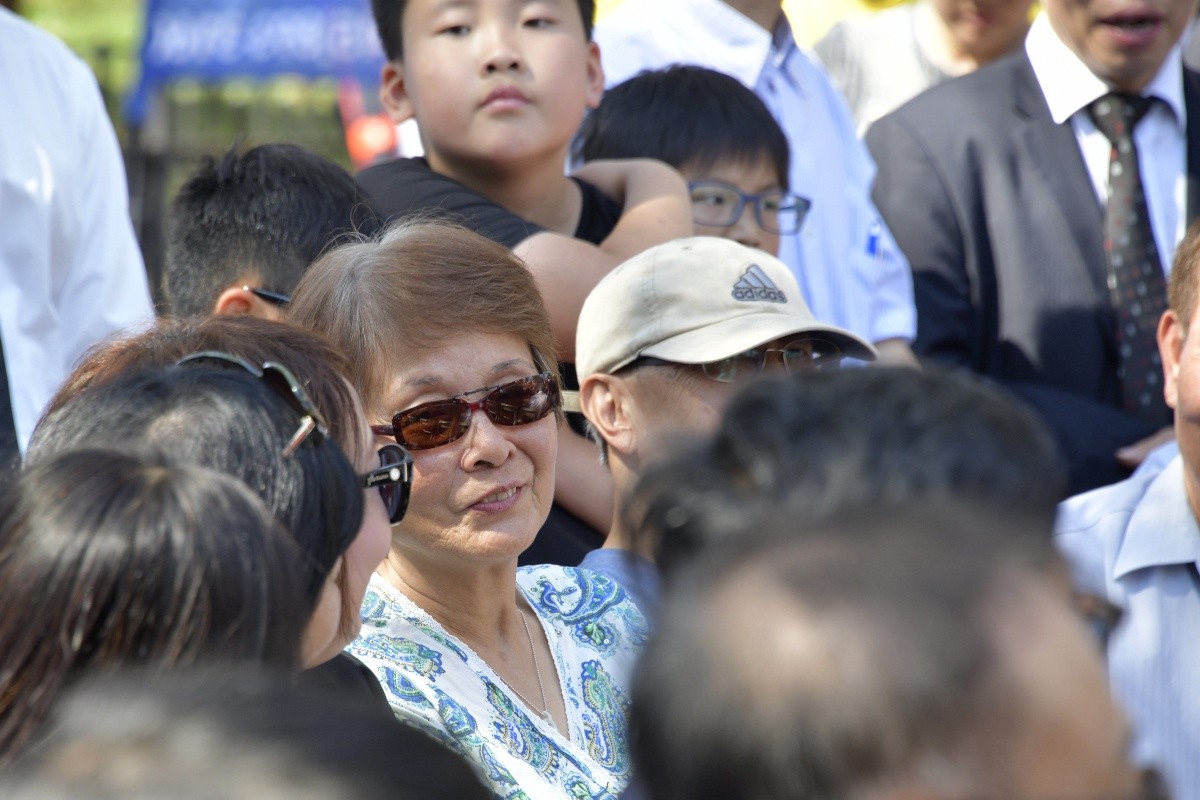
0 0 1200 800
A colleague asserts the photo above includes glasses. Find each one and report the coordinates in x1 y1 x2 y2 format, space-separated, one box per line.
175 349 331 456
356 443 415 528
689 178 811 236
629 337 848 386
365 371 563 452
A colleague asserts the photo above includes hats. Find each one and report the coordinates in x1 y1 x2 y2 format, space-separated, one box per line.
570 235 879 398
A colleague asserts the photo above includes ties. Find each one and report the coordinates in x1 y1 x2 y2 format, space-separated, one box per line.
1084 92 1175 431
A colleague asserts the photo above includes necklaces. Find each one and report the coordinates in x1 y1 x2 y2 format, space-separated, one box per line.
486 609 557 731
